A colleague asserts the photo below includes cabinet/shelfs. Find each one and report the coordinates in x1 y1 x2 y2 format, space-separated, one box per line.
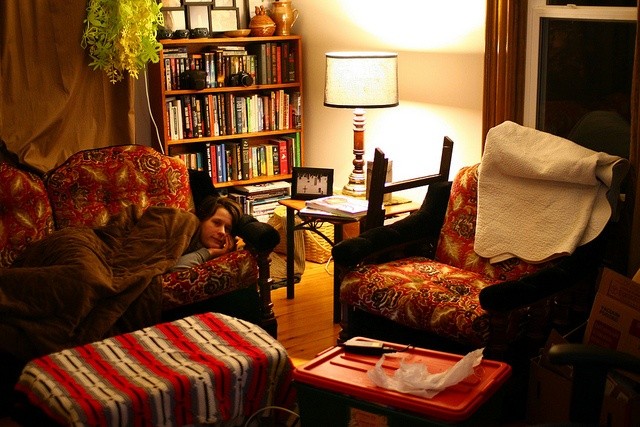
157 35 304 225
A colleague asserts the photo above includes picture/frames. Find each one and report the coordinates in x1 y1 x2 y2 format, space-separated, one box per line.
159 8 186 32
159 0 184 9
186 5 210 35
210 8 240 34
182 0 213 5
213 0 236 9
291 165 334 199
245 0 281 26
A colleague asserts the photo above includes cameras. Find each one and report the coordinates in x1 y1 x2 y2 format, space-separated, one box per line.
227 72 254 86
180 70 207 91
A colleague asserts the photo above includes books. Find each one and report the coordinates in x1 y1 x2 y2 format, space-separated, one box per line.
174 133 301 183
305 194 384 217
232 183 292 224
162 42 296 90
166 91 302 140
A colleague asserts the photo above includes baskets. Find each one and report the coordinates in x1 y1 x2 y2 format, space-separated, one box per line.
268 206 335 264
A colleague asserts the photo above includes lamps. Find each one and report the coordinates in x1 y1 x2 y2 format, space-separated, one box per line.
325 49 398 194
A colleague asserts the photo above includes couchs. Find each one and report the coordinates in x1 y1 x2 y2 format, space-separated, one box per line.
0 136 282 341
331 164 614 357
19 308 296 427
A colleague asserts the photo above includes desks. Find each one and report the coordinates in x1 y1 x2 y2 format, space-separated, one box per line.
278 191 424 303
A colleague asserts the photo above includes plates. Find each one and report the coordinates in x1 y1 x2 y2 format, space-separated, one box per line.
223 28 252 38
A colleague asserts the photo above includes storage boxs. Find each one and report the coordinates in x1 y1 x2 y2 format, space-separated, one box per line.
294 336 511 425
579 267 640 384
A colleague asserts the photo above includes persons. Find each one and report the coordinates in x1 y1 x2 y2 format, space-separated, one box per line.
0 198 243 353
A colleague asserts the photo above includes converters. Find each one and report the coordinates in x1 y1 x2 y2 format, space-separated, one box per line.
345 340 384 356
